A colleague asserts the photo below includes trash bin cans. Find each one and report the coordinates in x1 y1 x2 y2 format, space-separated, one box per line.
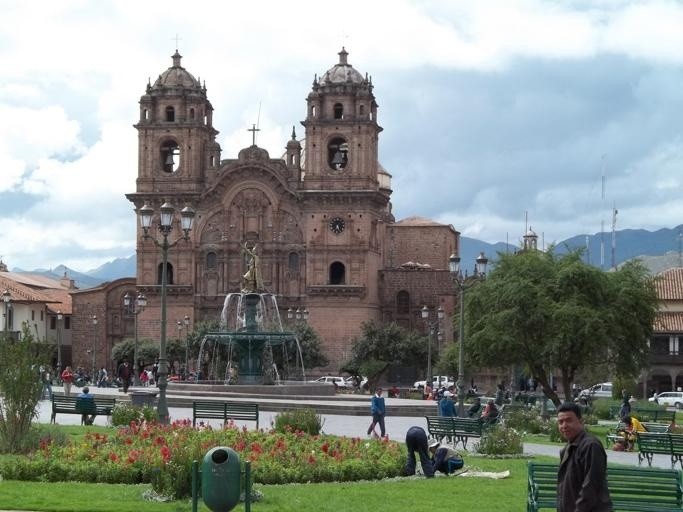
191 446 251 512
128 391 157 411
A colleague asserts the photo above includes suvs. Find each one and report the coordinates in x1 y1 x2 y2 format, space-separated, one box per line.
307 376 347 387
344 375 369 390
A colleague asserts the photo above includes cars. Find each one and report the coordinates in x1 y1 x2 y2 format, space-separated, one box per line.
28 363 54 380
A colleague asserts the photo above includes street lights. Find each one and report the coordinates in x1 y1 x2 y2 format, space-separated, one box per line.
135 196 197 425
86 348 90 381
56 310 62 378
447 251 488 397
91 314 96 385
176 320 182 380
123 291 147 386
183 315 189 383
1 286 11 342
422 304 445 390
286 307 309 380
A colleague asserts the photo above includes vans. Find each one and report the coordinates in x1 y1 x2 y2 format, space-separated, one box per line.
578 382 632 400
647 391 682 410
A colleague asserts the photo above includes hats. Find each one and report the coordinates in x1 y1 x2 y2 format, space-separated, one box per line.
427 438 440 451
444 390 454 397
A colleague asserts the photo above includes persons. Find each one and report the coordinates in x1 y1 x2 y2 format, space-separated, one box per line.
428 438 463 476
41 361 196 426
367 387 387 436
391 385 399 398
554 402 615 512
239 241 262 293
420 376 523 419
620 415 647 451
407 426 435 477
619 388 631 418
653 392 659 405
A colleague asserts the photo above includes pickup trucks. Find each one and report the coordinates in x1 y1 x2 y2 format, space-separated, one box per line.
413 375 456 389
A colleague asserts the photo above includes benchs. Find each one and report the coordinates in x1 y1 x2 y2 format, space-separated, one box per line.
191 401 260 430
605 421 672 452
635 429 683 468
50 393 116 425
424 414 486 451
498 405 531 419
635 409 676 424
525 461 683 512
